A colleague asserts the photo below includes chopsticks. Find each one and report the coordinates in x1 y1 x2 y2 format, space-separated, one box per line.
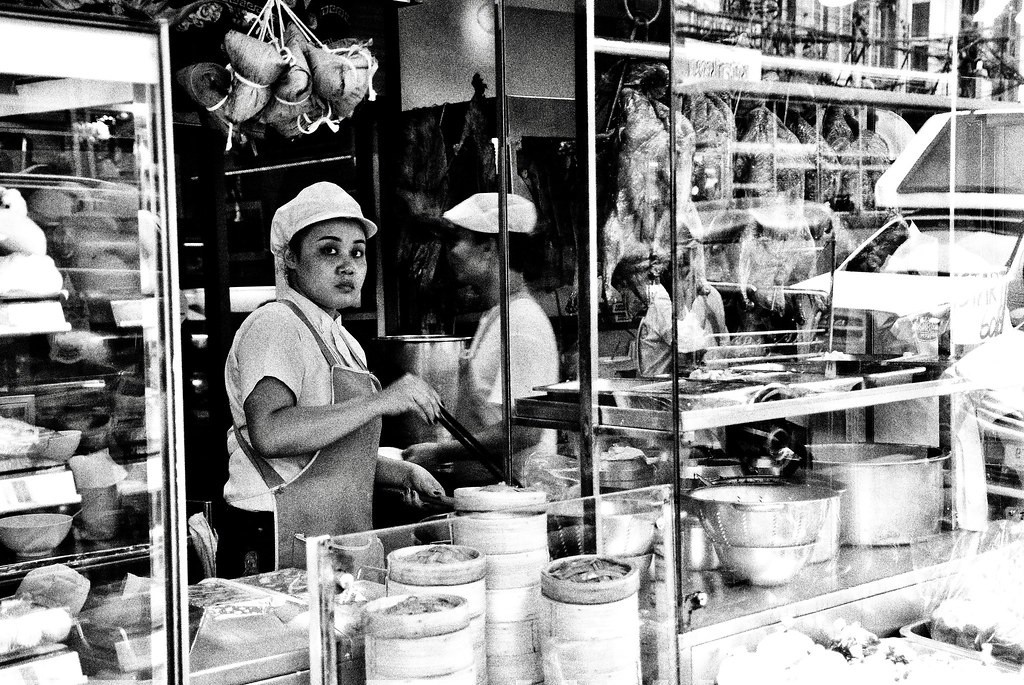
436 403 521 488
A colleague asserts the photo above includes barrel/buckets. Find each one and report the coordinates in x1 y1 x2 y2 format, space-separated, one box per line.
374 336 471 451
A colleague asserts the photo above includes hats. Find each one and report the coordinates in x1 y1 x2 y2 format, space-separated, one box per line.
269 181 378 259
443 192 538 233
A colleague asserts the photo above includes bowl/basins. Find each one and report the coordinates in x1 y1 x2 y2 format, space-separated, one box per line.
546 442 953 589
1 513 72 558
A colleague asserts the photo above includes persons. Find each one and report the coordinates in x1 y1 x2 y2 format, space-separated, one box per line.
401 193 558 488
611 255 730 458
785 295 829 468
215 182 445 580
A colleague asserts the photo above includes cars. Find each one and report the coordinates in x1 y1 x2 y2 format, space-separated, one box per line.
936 319 1024 510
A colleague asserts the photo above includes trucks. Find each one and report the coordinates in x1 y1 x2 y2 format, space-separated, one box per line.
781 108 1024 354
